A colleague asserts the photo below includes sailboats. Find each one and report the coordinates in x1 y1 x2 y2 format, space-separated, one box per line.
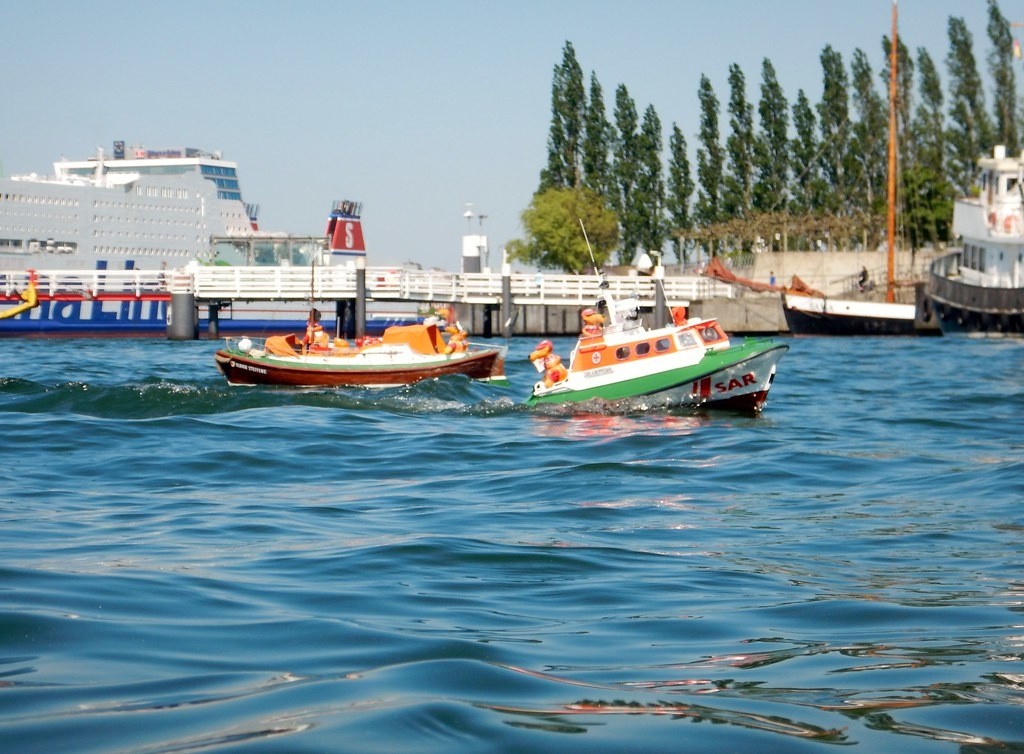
699 0 916 335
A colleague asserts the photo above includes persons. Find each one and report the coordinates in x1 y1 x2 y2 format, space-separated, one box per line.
858 265 869 288
769 271 776 286
299 319 311 355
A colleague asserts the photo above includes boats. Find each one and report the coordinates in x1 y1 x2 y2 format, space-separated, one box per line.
0 140 287 293
214 261 510 388
927 144 1024 339
527 218 789 415
